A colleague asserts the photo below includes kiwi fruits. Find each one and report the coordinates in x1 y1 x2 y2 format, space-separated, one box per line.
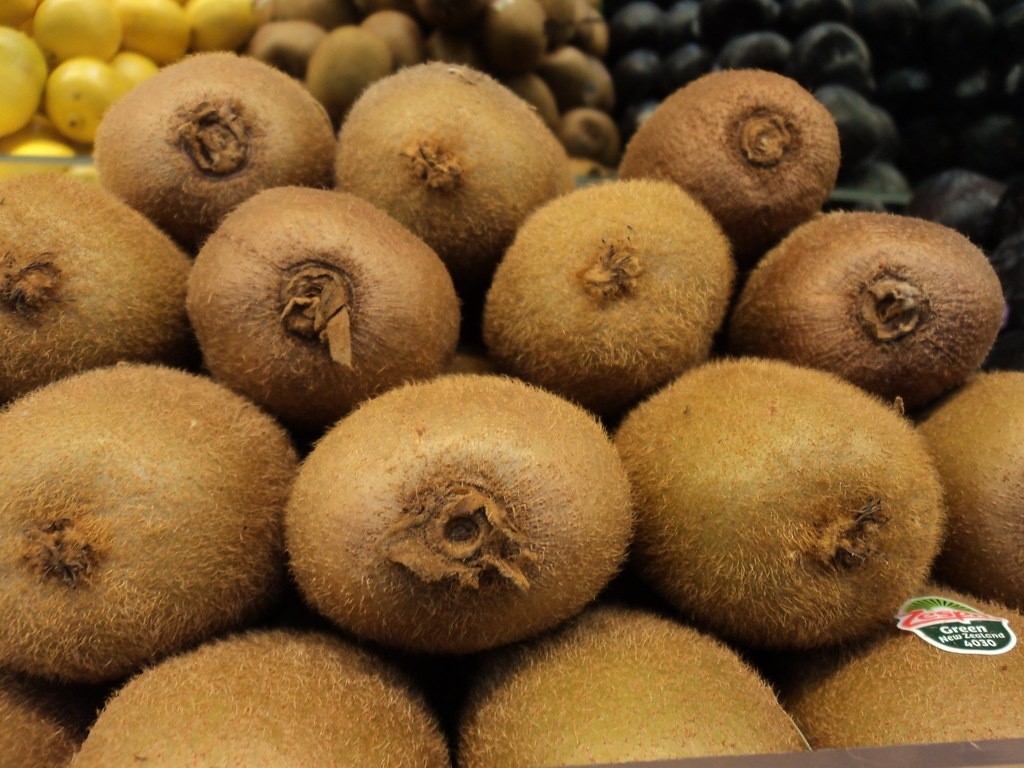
0 52 1024 768
248 1 621 185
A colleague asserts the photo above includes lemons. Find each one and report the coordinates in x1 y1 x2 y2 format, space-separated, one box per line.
0 0 273 157
